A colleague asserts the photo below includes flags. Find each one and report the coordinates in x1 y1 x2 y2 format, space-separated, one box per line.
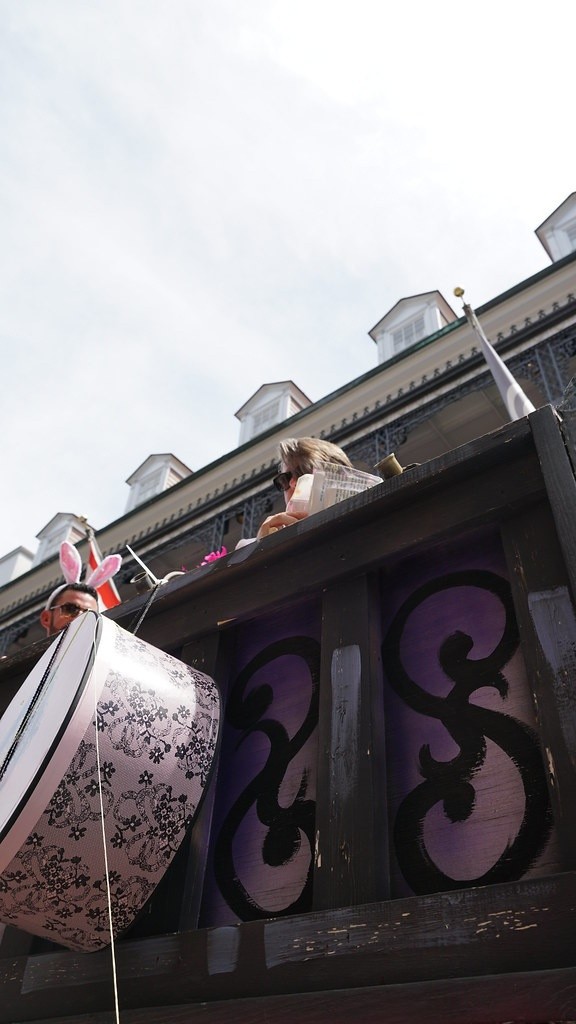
85 542 120 613
471 318 536 420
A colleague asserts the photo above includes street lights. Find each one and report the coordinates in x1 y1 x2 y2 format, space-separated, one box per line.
454 287 536 421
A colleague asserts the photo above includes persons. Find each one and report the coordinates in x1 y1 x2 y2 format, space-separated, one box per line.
257 438 353 537
41 584 99 637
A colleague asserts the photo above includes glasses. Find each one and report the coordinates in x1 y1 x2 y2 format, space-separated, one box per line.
50 603 98 620
273 464 313 492
